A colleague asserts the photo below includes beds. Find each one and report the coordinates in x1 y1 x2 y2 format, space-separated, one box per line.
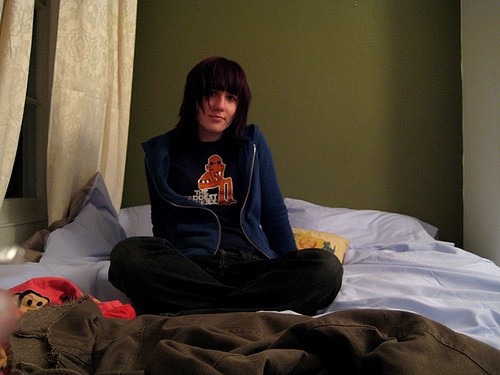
0 198 496 375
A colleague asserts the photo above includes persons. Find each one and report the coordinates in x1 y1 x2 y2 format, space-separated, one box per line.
109 56 344 316
